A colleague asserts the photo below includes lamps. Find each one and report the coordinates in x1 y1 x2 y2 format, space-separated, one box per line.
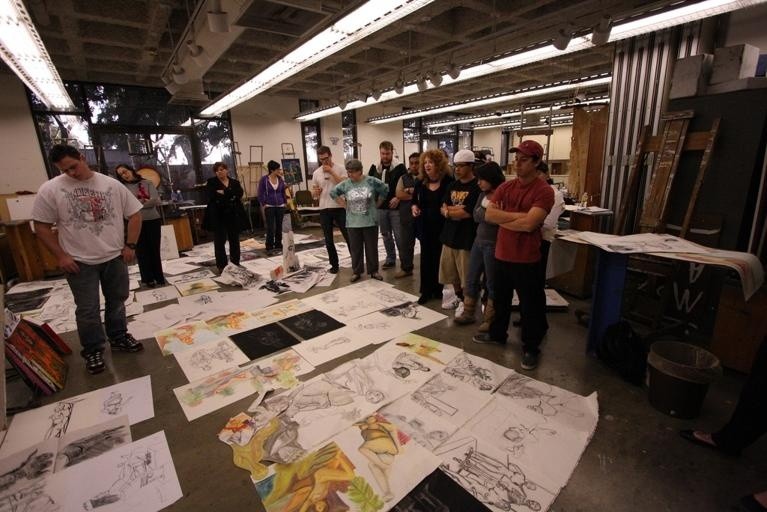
159 0 230 96
336 27 462 113
553 7 615 51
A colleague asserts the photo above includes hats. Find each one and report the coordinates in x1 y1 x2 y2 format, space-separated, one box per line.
510 140 543 155
454 150 475 163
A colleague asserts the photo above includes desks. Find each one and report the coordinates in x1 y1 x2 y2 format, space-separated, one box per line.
161 200 195 257
587 237 735 367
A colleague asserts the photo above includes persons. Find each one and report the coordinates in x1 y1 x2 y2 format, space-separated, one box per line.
257 160 288 256
310 146 351 273
367 140 408 269
115 164 165 288
471 140 556 371
328 159 389 282
31 144 143 375
395 147 565 345
201 162 252 274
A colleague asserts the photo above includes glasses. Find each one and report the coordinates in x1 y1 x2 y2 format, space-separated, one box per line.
320 154 330 161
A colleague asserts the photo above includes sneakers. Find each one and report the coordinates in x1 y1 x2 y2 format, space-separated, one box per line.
330 261 540 371
111 334 143 352
86 352 105 373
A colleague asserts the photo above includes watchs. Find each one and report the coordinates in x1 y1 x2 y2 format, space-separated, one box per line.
125 242 137 251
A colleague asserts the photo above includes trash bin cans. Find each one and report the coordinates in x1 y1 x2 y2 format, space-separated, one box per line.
646 341 720 417
